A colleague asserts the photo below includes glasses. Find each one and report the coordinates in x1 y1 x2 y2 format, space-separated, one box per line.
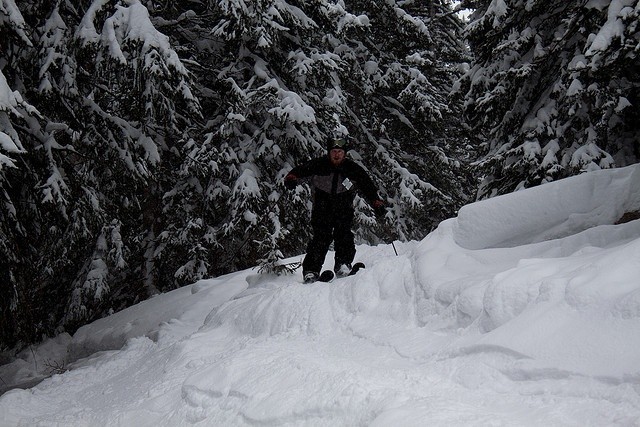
329 138 346 148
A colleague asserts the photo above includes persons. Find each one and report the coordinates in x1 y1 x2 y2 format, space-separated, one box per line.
283 136 388 283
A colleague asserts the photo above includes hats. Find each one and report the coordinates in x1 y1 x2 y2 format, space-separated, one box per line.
327 137 346 152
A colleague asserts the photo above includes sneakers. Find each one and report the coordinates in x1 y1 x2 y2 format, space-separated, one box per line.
303 271 318 283
336 263 352 278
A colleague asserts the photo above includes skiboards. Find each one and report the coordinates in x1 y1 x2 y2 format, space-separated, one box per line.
302 263 367 284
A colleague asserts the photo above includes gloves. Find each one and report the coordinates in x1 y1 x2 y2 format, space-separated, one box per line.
373 200 385 214
284 175 297 188
